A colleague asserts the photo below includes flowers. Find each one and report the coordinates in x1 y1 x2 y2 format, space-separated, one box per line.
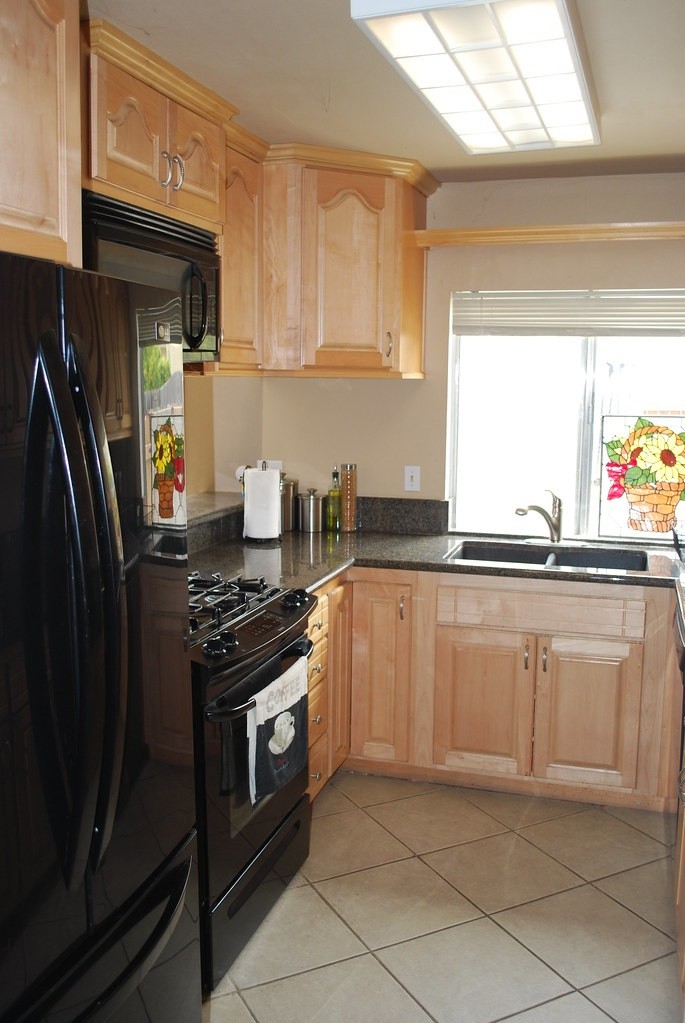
604 417 685 499
152 418 185 492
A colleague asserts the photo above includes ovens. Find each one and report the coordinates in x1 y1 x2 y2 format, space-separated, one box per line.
81 190 220 364
191 626 312 994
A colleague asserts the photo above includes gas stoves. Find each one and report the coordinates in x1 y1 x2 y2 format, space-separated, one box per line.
189 567 319 690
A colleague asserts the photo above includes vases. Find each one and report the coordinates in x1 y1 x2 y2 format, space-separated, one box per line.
155 474 175 518
621 482 685 532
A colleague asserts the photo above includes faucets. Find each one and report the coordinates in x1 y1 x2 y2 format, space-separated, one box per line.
514 489 562 542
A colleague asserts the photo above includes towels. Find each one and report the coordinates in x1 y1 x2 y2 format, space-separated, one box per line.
246 656 310 807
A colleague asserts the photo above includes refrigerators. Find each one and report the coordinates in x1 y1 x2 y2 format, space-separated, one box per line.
1 250 206 1023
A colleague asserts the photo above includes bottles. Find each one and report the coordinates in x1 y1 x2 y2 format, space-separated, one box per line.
340 463 357 532
326 471 341 532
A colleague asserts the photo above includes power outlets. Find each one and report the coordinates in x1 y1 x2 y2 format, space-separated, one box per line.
405 466 420 491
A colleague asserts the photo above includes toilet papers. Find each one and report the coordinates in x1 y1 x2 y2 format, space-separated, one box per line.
243 543 282 589
242 469 282 540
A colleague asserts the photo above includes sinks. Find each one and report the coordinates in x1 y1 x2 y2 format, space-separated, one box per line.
444 540 553 564
552 546 651 572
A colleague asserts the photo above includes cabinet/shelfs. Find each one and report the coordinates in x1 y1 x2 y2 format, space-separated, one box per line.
305 566 682 814
0 0 428 380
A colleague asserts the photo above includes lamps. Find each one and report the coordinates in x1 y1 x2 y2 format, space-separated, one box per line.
351 0 601 156
